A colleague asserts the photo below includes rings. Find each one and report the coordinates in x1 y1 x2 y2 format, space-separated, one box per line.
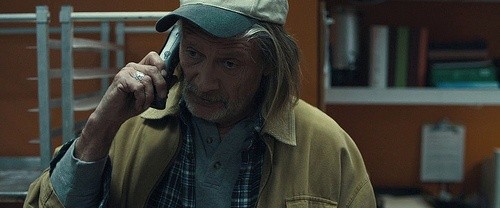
137 72 145 82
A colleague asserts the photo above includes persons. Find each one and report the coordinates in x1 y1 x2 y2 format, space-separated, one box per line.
22 0 377 208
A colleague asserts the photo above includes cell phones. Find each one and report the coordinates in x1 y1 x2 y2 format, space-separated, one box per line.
150 25 180 110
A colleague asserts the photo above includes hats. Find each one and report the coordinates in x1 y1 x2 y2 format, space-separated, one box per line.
155 0 289 38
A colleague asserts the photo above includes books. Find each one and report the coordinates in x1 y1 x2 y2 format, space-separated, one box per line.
366 24 500 89
